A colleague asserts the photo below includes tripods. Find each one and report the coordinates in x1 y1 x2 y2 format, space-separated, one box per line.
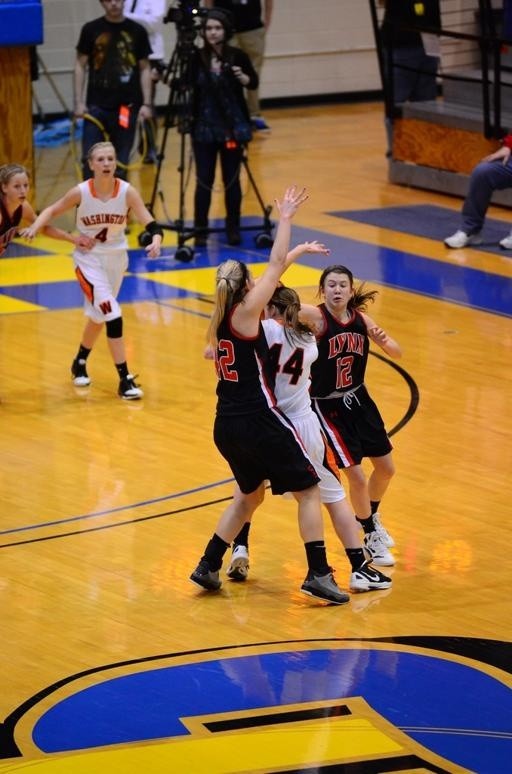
137 42 274 263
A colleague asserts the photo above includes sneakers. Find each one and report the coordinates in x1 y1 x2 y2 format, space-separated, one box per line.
349 512 394 590
300 566 351 605
118 373 145 400
499 230 512 250
444 228 484 248
225 545 251 581
71 358 91 386
190 559 222 591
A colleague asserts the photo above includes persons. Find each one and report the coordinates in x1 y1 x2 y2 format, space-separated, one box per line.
0 162 95 253
442 135 511 252
204 0 273 134
74 0 154 234
299 265 401 567
371 0 442 158
188 183 350 604
202 286 392 593
123 0 165 164
178 11 260 248
20 142 163 402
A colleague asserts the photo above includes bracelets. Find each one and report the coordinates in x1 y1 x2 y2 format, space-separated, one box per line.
144 103 151 107
